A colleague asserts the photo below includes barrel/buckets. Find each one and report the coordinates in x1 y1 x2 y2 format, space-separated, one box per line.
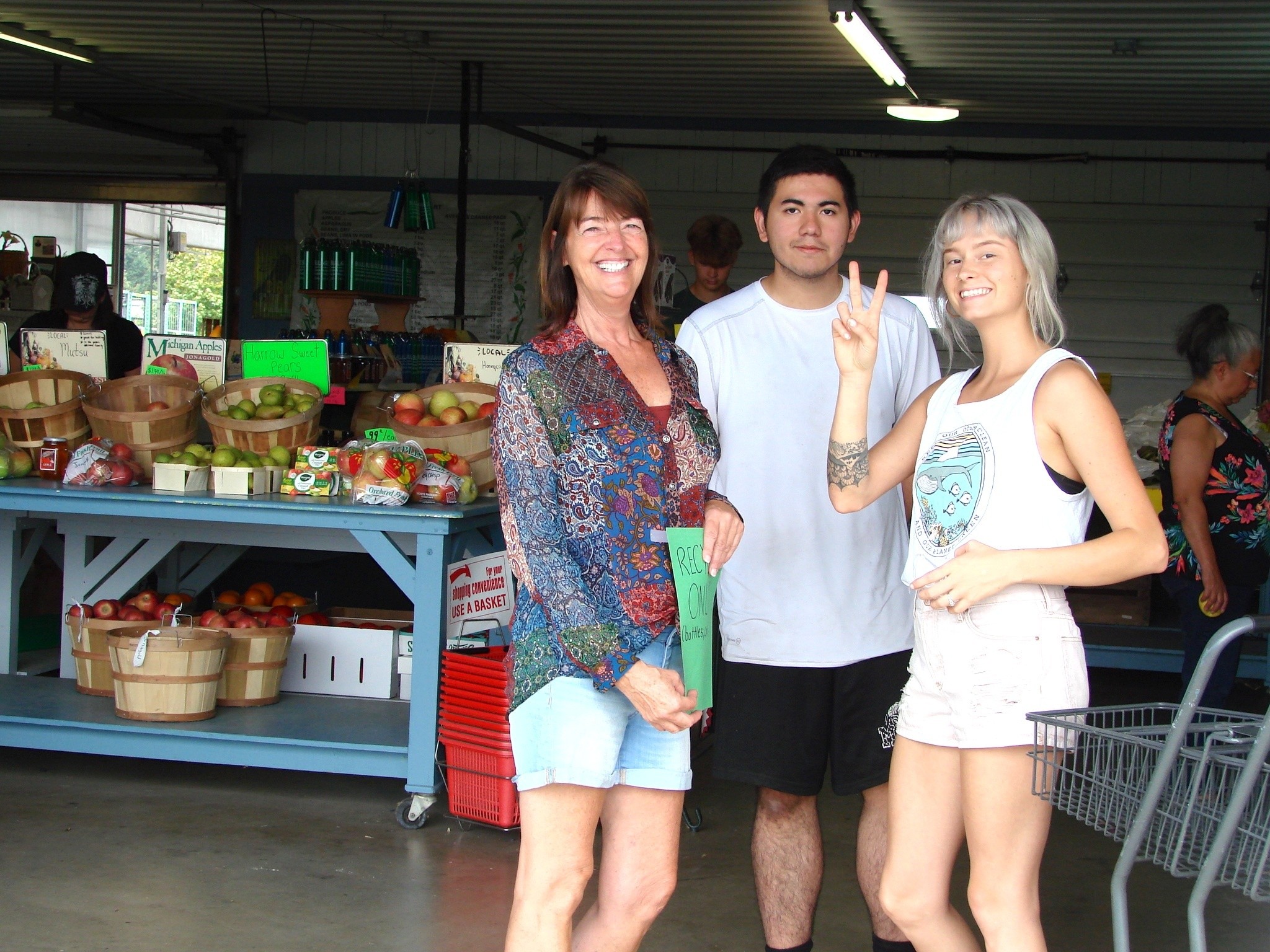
0 370 94 476
65 604 181 698
197 375 324 468
77 374 206 483
106 615 232 722
213 590 318 624
181 611 297 707
378 382 498 493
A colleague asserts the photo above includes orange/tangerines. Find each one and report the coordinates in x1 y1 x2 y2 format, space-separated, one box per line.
159 582 308 606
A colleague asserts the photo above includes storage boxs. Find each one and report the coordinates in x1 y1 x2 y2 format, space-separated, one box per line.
279 606 487 701
152 447 343 498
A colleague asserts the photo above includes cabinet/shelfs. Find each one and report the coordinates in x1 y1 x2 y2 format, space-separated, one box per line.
1075 578 1270 697
297 289 420 404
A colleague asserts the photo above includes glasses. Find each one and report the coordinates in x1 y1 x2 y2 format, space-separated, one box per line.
1240 360 1260 383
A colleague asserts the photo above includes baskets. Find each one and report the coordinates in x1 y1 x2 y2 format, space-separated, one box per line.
439 618 521 827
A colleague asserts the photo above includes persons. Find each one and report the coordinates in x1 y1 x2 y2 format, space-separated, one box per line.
652 214 743 343
488 162 745 952
827 191 1170 952
675 144 942 952
8 252 143 380
1159 302 1270 803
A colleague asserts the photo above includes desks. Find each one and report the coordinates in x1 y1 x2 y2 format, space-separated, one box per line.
0 476 504 829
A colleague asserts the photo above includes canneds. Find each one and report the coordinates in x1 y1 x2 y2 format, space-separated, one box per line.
39 437 69 480
329 354 393 384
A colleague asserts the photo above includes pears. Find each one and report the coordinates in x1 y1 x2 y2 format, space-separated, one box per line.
214 383 317 420
154 445 291 493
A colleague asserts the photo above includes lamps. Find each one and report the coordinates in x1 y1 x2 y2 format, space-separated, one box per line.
0 24 95 63
827 1 959 122
1248 270 1263 301
1056 263 1068 293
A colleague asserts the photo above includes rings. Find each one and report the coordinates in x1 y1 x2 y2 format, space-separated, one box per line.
948 592 955 607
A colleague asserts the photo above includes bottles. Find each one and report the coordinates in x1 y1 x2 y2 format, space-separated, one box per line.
39 437 69 480
298 234 423 300
385 169 436 232
272 329 446 382
319 429 357 448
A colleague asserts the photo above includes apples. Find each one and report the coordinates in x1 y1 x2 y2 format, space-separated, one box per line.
146 355 198 382
392 390 497 427
0 432 32 480
66 436 144 486
294 612 394 630
1199 591 1223 617
146 401 168 412
1 400 49 409
67 590 296 629
336 440 479 505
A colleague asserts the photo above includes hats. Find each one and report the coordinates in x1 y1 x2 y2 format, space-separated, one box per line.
57 251 107 314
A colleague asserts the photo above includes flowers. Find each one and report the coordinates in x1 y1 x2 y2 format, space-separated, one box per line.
0 232 18 250
